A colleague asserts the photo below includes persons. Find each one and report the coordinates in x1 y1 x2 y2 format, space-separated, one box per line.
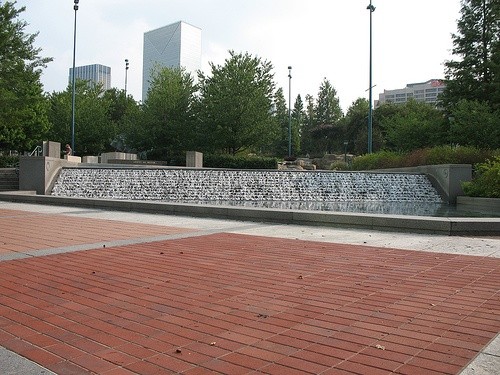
66 144 72 156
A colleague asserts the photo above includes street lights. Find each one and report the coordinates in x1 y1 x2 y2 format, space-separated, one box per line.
367 0 376 152
71 0 78 153
287 65 292 157
124 60 129 97
